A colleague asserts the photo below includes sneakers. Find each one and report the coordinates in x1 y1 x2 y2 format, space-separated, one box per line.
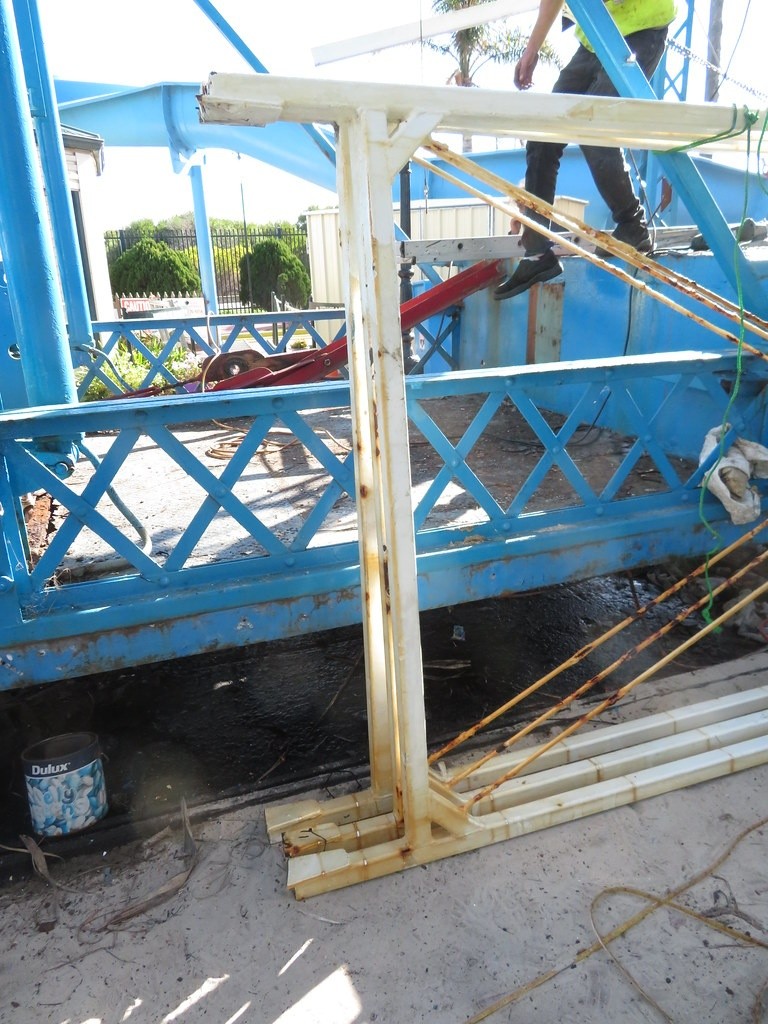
595 221 652 255
494 249 562 300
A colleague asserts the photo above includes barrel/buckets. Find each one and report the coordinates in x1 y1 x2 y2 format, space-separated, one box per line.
21 731 109 838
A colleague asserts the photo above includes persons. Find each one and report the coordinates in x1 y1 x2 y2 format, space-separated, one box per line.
492 0 677 301
508 217 521 235
